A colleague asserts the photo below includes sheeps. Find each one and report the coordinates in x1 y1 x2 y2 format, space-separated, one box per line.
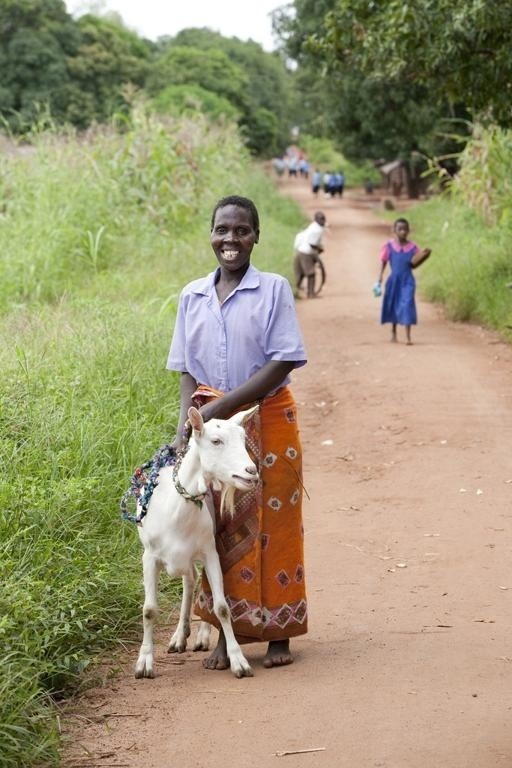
134 404 260 680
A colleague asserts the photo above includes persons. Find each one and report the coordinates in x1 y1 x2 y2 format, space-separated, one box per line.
375 217 432 344
165 195 307 669
293 213 327 297
268 154 346 200
